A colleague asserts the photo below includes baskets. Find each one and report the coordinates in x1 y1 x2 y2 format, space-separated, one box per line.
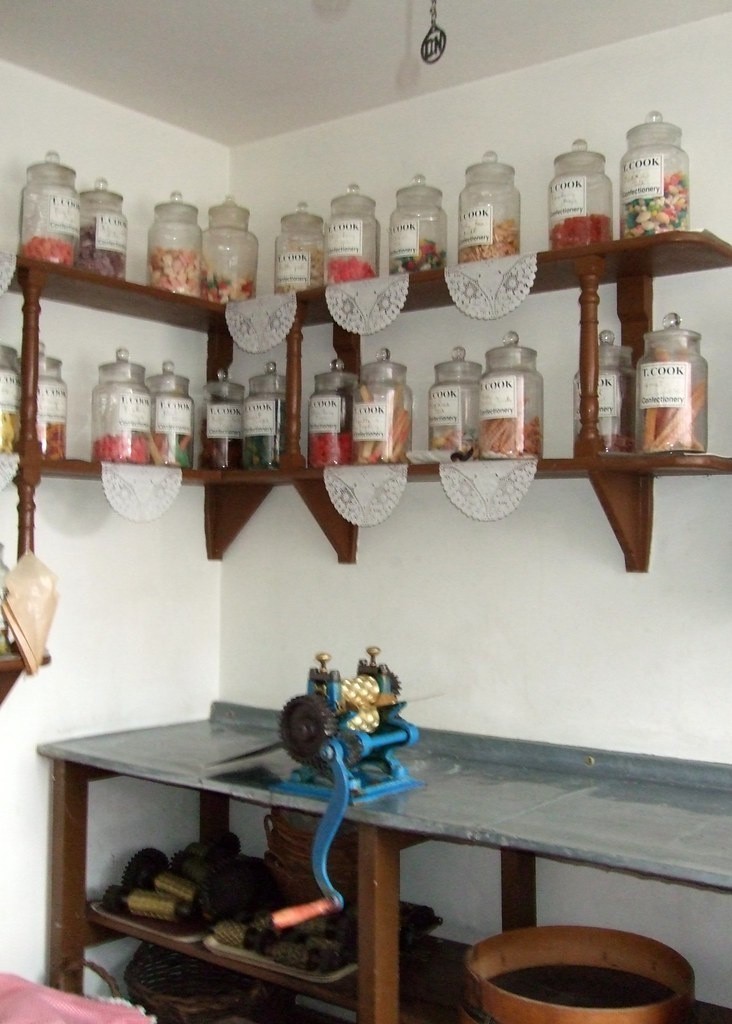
263 806 359 932
123 940 278 1024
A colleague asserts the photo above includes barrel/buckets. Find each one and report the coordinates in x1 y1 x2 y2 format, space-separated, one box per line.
454 923 697 1024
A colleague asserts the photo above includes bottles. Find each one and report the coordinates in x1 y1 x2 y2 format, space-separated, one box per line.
18 151 129 283
426 331 545 463
458 151 522 264
389 175 447 276
572 312 710 457
0 341 67 464
547 138 613 253
146 191 259 306
306 346 412 471
619 111 692 240
86 347 198 472
275 183 379 298
196 360 288 473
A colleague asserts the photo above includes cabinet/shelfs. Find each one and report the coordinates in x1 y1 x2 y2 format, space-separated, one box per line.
37 701 732 1024
8 232 732 573
